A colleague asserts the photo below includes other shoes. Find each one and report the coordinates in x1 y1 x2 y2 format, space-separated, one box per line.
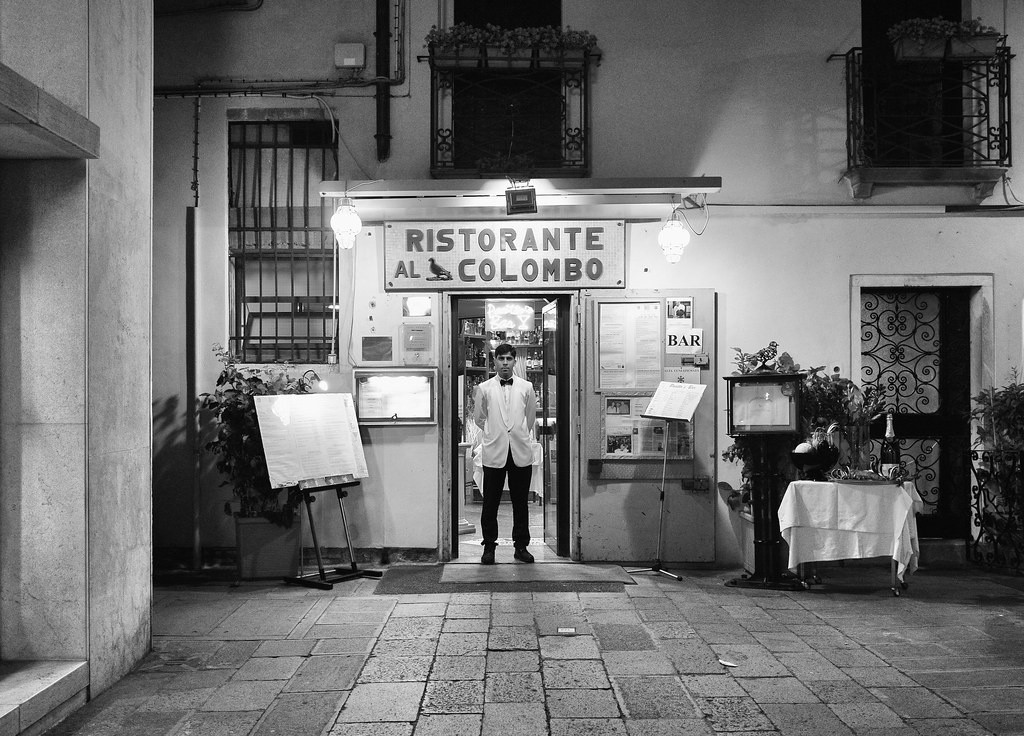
481 544 495 564
514 547 534 562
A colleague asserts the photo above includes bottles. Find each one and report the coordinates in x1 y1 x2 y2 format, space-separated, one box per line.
464 320 542 406
882 414 901 480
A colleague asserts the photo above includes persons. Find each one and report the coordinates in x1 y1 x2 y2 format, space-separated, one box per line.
474 343 535 564
657 442 663 451
673 301 685 318
618 401 628 414
608 436 630 452
607 402 616 413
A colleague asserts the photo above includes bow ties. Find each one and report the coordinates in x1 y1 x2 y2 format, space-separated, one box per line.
500 378 513 386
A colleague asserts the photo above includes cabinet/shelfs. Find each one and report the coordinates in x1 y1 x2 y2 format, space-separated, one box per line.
721 373 810 591
776 478 919 597
459 331 543 417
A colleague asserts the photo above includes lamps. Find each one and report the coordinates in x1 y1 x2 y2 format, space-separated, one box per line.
330 180 362 249
658 195 690 264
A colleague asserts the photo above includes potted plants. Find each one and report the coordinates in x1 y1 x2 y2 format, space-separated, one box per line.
885 17 955 60
198 341 320 581
424 22 598 70
946 15 1000 58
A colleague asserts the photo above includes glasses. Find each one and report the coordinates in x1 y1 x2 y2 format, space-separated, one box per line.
496 357 515 362
